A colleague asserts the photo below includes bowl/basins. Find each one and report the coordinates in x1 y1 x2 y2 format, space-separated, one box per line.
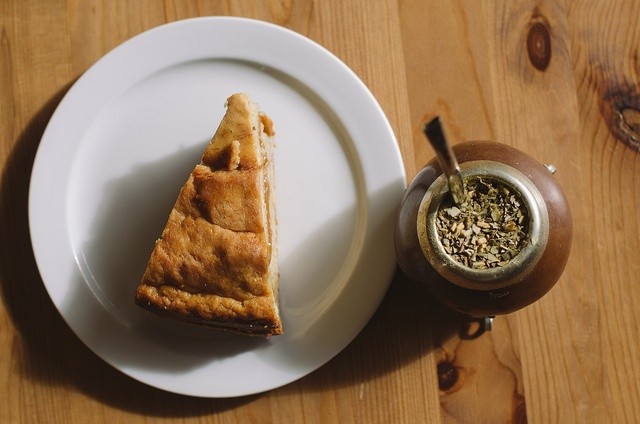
393 139 573 317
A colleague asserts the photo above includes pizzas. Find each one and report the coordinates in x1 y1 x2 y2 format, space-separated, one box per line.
133 92 284 338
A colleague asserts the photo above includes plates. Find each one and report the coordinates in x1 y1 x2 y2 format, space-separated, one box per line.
27 14 410 400
